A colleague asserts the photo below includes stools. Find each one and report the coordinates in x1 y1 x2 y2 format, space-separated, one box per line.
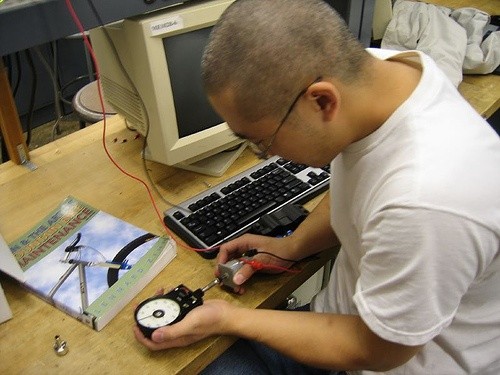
56 31 94 120
74 80 120 130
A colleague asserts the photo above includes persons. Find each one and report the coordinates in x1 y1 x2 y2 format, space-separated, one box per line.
129 0 500 374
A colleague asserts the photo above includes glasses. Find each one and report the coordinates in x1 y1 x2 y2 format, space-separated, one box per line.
246 76 324 160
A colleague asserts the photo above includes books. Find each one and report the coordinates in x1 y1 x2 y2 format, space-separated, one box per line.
8 195 177 332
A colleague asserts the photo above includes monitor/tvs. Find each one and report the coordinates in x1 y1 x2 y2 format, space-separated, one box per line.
88 0 243 166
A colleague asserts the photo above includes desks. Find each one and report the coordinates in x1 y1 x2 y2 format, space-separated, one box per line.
0 72 500 375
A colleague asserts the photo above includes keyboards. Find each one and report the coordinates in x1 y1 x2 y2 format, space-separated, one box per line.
164 153 330 259
